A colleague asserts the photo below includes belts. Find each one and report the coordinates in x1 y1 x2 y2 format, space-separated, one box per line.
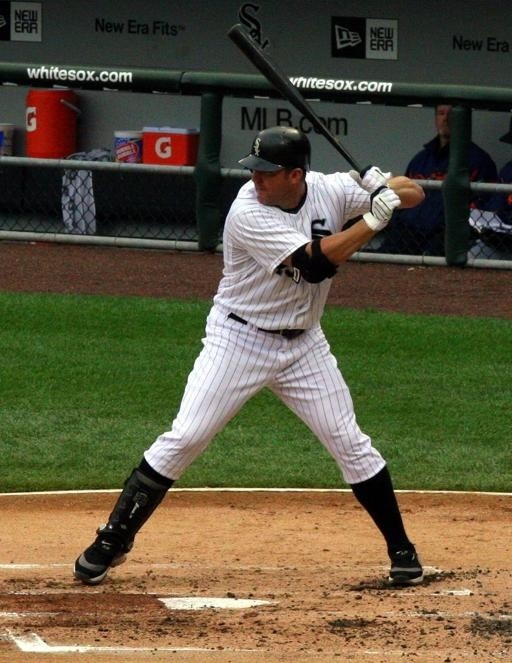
227 312 304 340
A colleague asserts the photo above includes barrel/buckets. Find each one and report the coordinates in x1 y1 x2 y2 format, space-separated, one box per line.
26 88 80 159
0 123 19 156
113 130 142 163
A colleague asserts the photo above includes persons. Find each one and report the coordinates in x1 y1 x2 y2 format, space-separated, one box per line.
74 128 427 585
467 117 512 260
376 102 505 255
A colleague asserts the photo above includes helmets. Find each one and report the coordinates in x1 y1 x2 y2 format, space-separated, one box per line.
238 126 311 173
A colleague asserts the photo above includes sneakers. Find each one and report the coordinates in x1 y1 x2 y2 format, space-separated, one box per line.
389 545 424 585
73 523 128 585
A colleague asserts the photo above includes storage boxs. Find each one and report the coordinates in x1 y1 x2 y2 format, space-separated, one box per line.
141 125 200 165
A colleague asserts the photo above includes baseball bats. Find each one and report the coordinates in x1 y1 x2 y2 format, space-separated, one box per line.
227 23 365 176
